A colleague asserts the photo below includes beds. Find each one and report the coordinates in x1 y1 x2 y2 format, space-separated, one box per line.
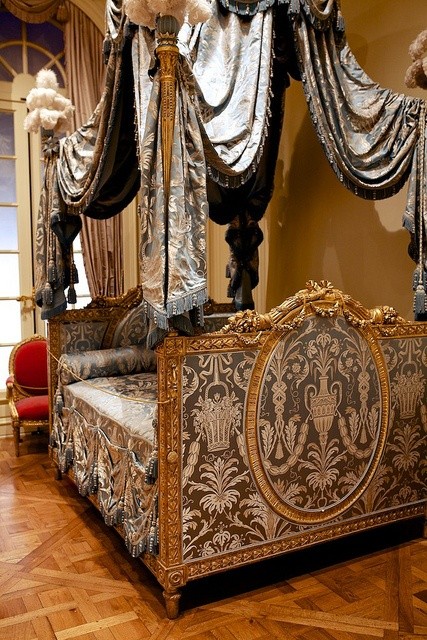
25 0 427 620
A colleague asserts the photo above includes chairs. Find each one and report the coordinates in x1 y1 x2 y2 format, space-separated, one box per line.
5 334 47 458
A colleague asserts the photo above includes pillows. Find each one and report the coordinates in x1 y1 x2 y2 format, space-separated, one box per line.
55 345 156 386
